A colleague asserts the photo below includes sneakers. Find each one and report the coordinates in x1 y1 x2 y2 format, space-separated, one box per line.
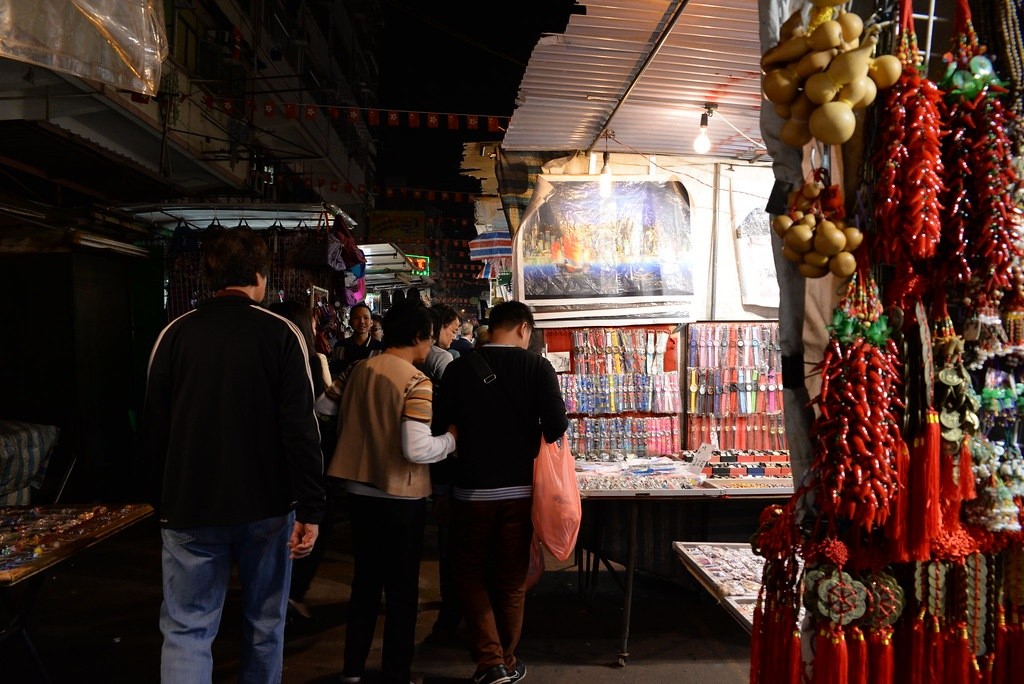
471 663 511 684
508 659 528 684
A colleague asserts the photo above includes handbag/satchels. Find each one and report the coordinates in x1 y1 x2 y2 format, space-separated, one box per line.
531 431 582 561
298 211 366 307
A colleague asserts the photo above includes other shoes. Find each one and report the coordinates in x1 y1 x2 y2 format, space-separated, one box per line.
336 671 361 684
411 671 425 684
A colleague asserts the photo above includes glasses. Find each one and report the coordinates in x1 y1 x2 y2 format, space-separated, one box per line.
430 334 438 347
444 324 459 337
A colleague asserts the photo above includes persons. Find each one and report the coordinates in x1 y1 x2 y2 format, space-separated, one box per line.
272 286 567 684
143 224 327 684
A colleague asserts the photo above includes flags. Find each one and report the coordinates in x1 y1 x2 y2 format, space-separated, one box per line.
142 94 511 132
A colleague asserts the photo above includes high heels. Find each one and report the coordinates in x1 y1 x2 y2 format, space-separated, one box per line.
286 600 327 630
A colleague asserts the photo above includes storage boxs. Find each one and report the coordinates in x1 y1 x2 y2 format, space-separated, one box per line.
678 450 793 477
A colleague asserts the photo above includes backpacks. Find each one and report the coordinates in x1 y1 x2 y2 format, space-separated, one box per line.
167 218 286 274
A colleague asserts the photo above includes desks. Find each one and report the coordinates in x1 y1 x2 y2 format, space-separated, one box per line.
671 539 803 639
0 503 152 683
574 494 788 666
